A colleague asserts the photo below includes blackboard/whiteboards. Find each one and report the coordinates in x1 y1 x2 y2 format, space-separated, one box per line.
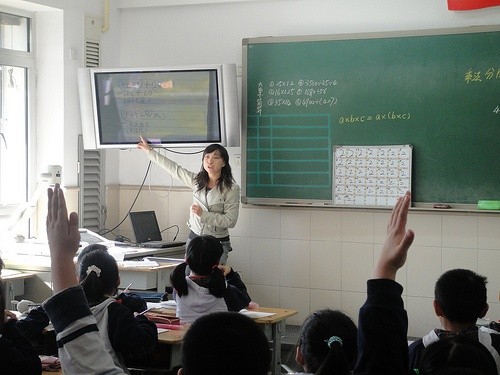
332 144 413 207
240 24 500 214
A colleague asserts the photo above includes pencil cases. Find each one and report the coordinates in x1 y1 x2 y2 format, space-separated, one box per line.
143 312 181 325
124 292 166 303
40 355 61 372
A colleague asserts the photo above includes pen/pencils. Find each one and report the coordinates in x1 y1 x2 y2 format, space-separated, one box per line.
123 283 134 291
140 308 153 315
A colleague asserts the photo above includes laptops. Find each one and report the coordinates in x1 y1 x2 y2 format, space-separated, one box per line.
129 211 186 248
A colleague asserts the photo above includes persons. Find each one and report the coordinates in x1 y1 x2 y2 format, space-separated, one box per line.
169 235 252 326
0 256 50 375
359 190 416 375
136 134 241 275
77 244 148 312
295 308 358 375
78 251 158 375
408 269 500 375
41 182 273 375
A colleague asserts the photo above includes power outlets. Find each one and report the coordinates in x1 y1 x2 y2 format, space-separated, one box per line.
234 154 241 166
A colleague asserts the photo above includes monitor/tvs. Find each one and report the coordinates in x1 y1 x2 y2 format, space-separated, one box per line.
77 64 240 151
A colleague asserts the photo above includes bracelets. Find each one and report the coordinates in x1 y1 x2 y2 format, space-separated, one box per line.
148 147 153 153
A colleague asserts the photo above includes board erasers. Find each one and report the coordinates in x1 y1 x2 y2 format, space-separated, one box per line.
477 199 500 209
433 203 449 209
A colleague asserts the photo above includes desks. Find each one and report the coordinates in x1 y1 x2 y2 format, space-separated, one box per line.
0 246 299 375
